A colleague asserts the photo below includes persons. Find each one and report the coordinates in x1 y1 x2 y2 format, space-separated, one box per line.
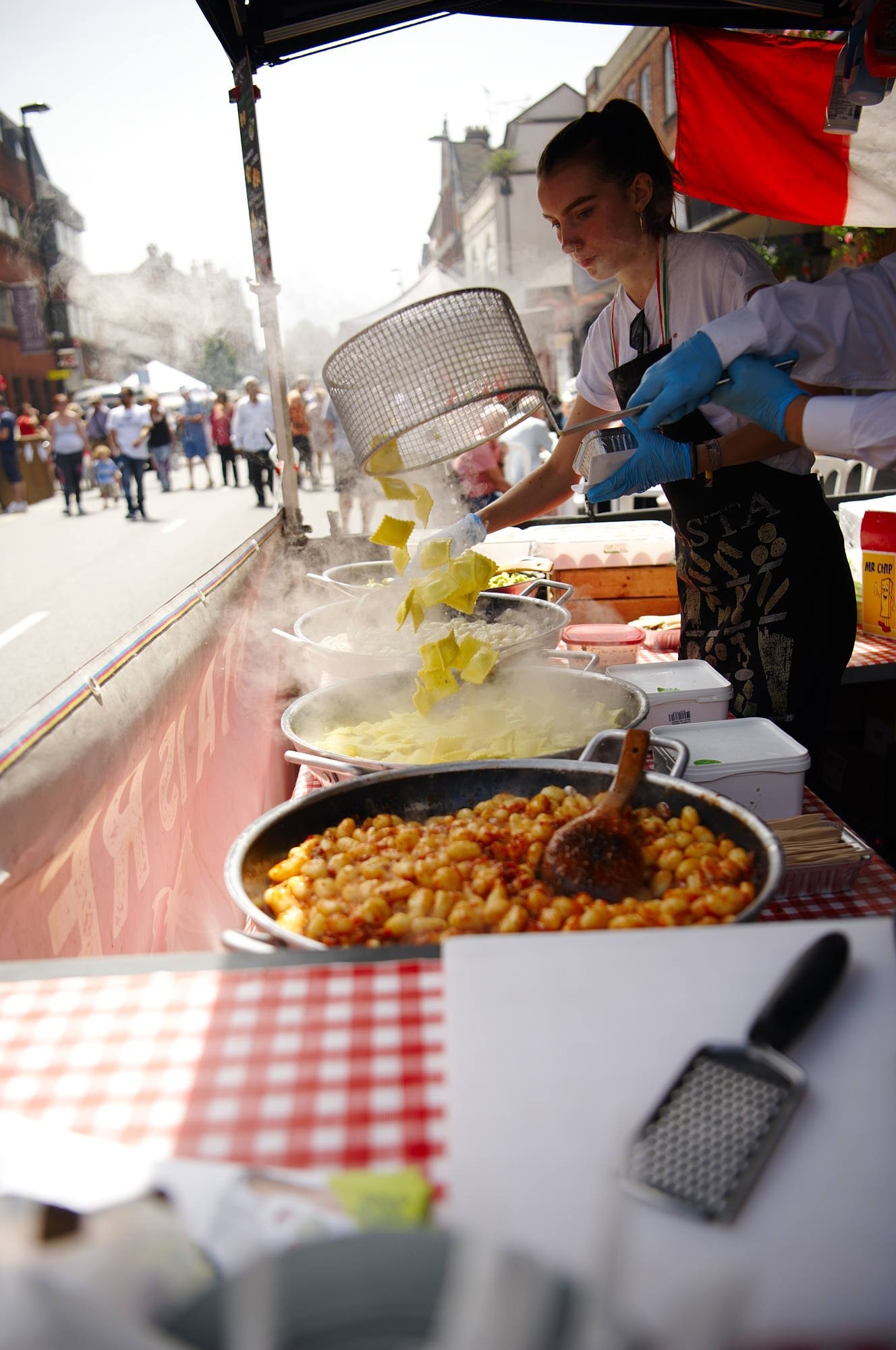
622 251 896 471
0 376 577 536
402 100 858 788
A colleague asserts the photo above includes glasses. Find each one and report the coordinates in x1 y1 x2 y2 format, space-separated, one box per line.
118 393 128 400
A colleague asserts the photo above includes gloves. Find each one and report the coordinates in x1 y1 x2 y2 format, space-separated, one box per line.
626 330 723 431
709 349 815 443
405 514 488 572
586 416 696 504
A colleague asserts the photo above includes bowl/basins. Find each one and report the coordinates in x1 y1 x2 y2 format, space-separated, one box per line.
572 423 666 482
564 624 645 671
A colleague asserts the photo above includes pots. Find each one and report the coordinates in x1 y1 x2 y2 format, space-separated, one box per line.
220 557 783 956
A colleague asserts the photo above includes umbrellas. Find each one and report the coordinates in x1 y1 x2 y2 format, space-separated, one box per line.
74 360 218 403
